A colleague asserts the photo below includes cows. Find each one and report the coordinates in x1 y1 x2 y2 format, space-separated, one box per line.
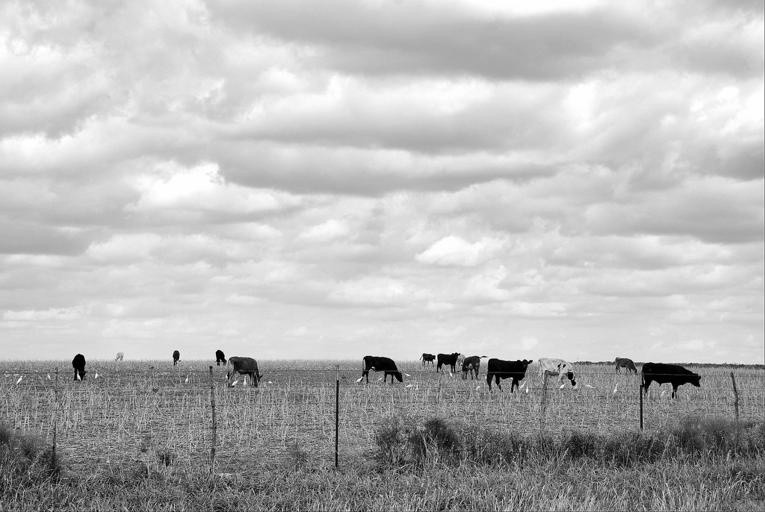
486 358 533 393
71 353 87 382
225 356 264 388
356 355 403 384
216 349 227 366
115 352 124 361
419 352 436 364
172 350 180 366
536 357 577 387
614 356 637 376
436 351 487 380
641 362 702 398
